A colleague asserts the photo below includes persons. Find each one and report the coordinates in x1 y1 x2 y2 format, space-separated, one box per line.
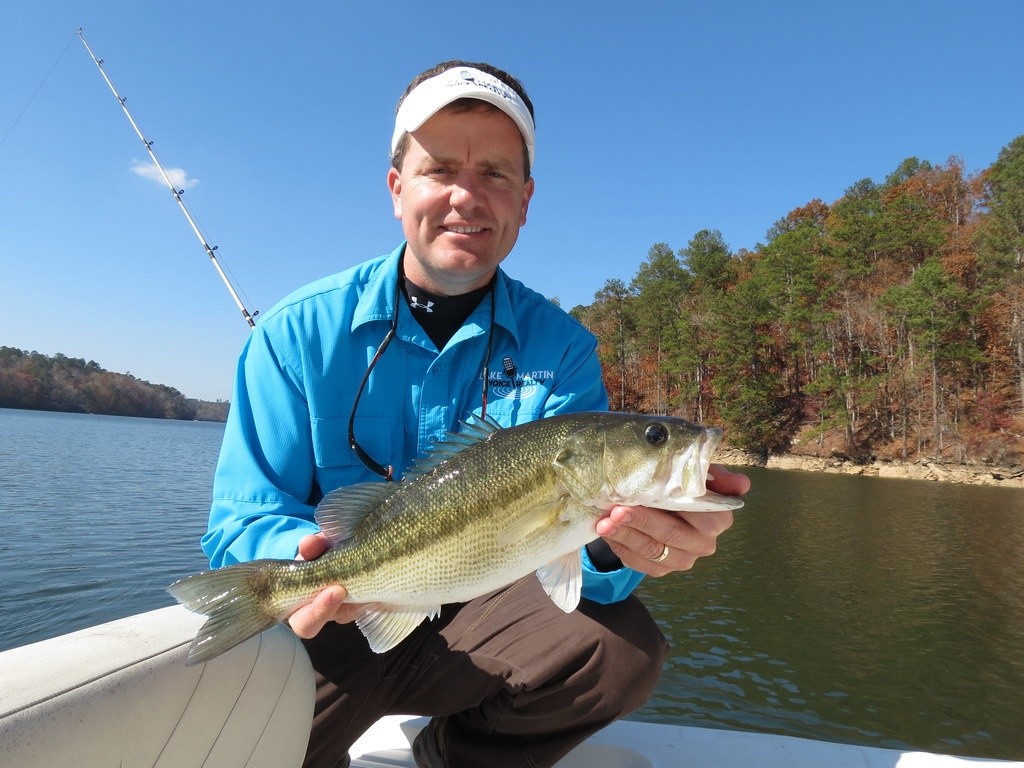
200 61 751 768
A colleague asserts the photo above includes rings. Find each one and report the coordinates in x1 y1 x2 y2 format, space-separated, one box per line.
648 544 669 563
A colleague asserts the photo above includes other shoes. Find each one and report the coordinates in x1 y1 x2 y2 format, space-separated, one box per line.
413 717 447 768
336 753 350 768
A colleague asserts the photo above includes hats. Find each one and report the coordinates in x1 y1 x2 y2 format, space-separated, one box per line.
392 66 534 177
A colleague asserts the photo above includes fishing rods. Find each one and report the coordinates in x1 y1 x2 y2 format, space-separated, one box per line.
77 25 260 333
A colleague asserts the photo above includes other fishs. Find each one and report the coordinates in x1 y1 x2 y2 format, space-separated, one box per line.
163 410 745 668
